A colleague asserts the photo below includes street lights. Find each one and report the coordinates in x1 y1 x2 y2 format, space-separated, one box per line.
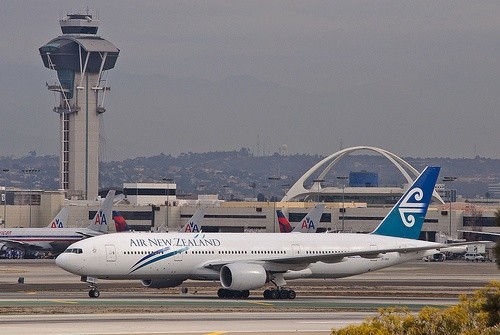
163 177 174 232
268 176 280 233
313 179 324 203
441 177 458 236
22 168 41 227
337 177 349 231
2 168 10 227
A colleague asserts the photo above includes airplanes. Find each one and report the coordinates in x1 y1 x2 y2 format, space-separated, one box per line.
0 190 116 258
54 166 493 298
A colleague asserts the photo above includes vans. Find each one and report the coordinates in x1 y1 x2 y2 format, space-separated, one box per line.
465 253 484 262
